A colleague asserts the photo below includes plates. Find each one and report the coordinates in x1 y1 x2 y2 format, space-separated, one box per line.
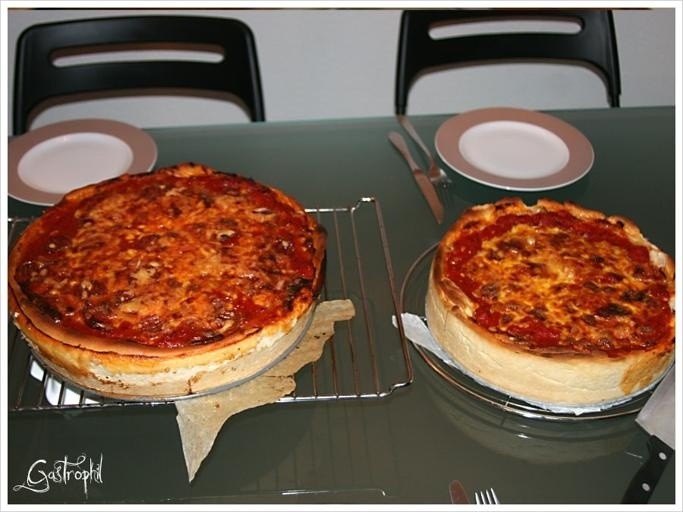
8 119 159 209
434 107 596 192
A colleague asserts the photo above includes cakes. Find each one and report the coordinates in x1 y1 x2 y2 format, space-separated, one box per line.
7 161 329 400
424 195 676 414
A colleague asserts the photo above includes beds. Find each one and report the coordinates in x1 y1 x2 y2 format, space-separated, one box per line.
5 104 675 501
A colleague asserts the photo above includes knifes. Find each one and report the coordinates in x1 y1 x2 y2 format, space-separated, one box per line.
619 363 674 503
386 130 446 225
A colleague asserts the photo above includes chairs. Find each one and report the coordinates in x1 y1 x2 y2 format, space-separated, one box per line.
391 10 624 117
13 15 265 136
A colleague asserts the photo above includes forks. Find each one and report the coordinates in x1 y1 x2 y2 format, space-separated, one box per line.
474 488 500 504
396 113 456 212
